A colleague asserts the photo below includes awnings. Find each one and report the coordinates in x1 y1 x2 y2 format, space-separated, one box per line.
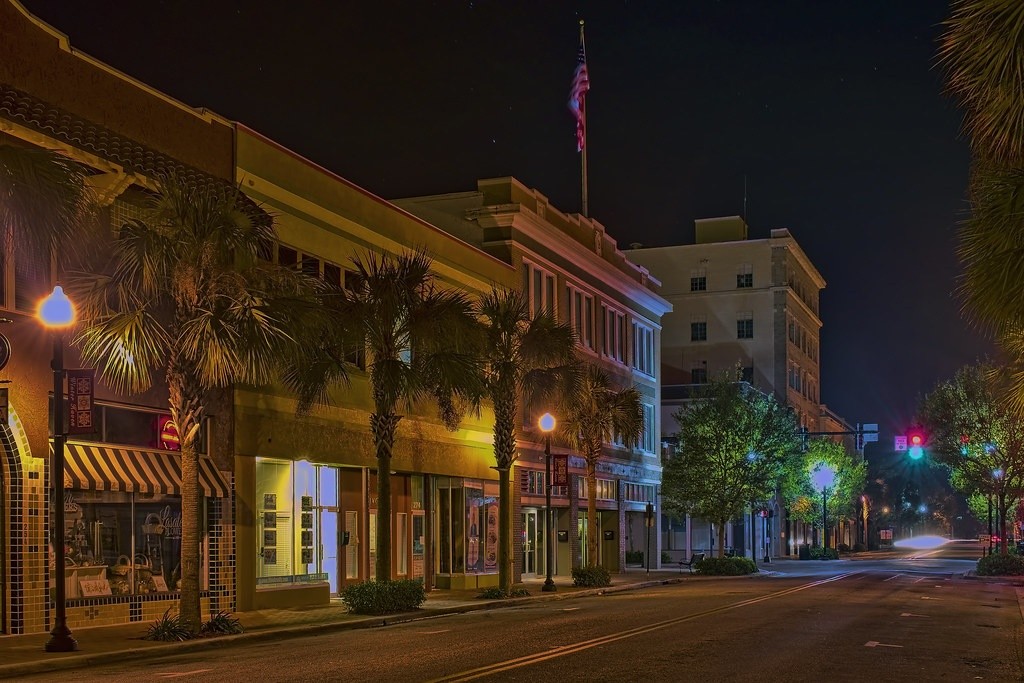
48 440 231 497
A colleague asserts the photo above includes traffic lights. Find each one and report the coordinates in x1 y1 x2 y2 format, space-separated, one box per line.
907 428 923 464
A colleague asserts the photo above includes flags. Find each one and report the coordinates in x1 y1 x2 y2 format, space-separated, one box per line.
570 24 589 152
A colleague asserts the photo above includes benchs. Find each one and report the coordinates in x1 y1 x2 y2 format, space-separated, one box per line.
678 553 705 572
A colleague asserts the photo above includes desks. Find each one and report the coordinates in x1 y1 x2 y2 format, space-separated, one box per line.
66 564 109 598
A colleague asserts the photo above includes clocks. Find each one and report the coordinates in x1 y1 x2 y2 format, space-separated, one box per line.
96 508 118 528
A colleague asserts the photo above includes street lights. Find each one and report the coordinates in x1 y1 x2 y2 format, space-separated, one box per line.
814 469 832 552
33 286 78 653
537 412 557 592
